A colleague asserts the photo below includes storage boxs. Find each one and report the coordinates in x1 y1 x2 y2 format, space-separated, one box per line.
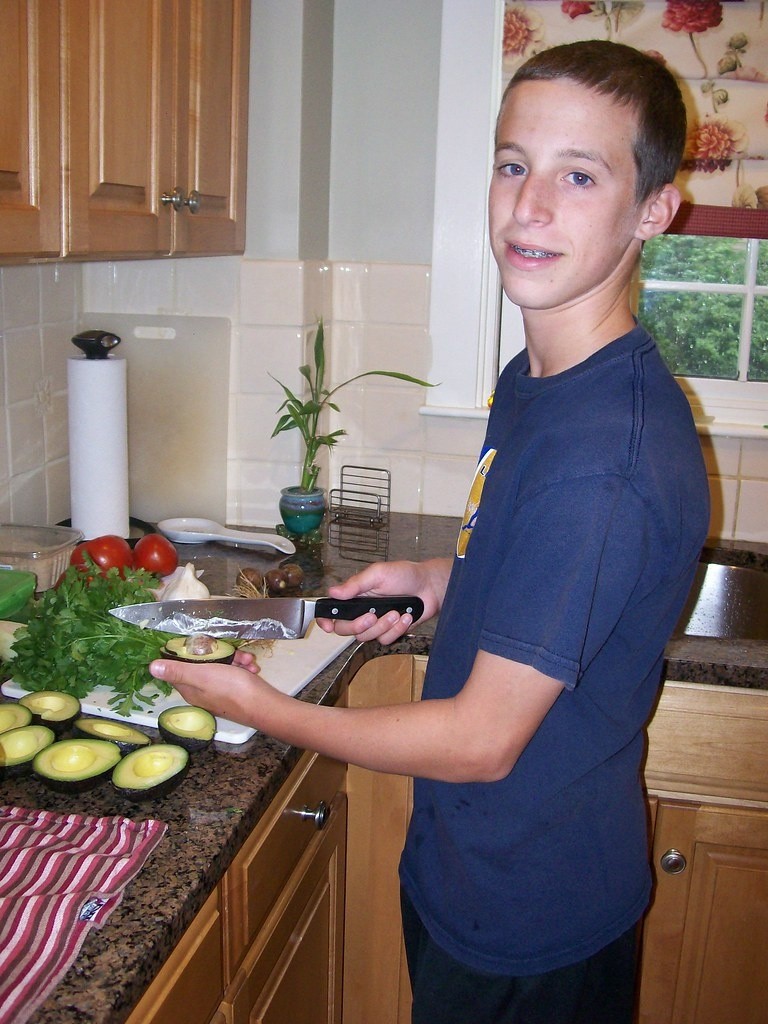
0 522 85 593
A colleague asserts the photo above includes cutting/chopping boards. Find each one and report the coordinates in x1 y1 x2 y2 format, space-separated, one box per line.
0 595 357 744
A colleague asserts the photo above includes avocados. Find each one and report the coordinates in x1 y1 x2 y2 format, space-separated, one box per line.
160 634 236 664
0 691 217 800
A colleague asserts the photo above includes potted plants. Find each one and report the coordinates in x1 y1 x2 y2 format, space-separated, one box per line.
266 314 444 545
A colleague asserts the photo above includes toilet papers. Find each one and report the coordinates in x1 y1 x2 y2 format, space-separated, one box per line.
65 349 130 540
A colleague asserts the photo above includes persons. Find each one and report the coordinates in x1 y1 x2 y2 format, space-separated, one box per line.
148 38 715 1023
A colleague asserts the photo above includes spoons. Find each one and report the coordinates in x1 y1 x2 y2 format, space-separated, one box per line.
157 518 296 554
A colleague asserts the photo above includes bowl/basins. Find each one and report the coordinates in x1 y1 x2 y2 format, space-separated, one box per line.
0 520 83 590
678 547 768 637
0 569 36 620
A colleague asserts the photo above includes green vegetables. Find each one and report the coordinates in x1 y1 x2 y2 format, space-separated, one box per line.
0 550 240 718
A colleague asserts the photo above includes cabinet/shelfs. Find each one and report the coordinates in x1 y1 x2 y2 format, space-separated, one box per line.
60 0 252 264
396 654 768 1024
0 0 64 268
124 750 348 1024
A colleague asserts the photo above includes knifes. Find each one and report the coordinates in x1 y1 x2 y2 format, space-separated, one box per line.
108 597 424 640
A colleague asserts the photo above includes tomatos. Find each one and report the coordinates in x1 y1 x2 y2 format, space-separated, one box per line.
56 534 178 589
